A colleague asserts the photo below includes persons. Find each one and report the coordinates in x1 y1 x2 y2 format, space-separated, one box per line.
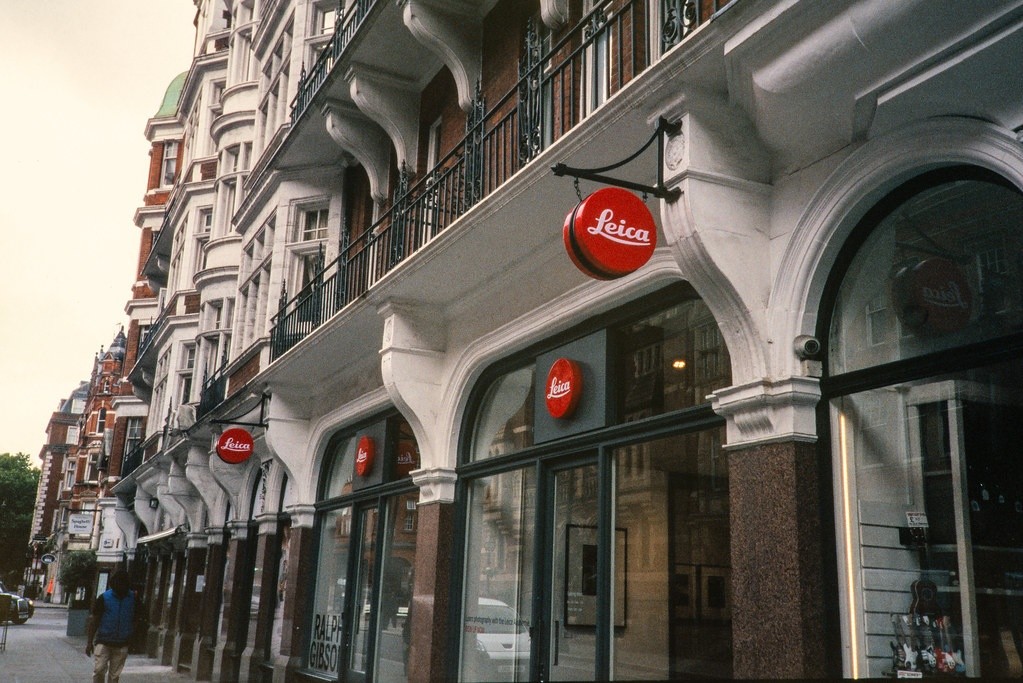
367 568 399 630
85 569 144 683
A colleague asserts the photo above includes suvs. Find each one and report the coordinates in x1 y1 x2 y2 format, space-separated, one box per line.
0 581 34 625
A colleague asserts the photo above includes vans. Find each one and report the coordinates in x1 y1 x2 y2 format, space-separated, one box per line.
250 583 261 616
477 598 533 680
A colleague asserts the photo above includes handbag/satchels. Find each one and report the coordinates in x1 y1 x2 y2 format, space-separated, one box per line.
130 590 150 654
